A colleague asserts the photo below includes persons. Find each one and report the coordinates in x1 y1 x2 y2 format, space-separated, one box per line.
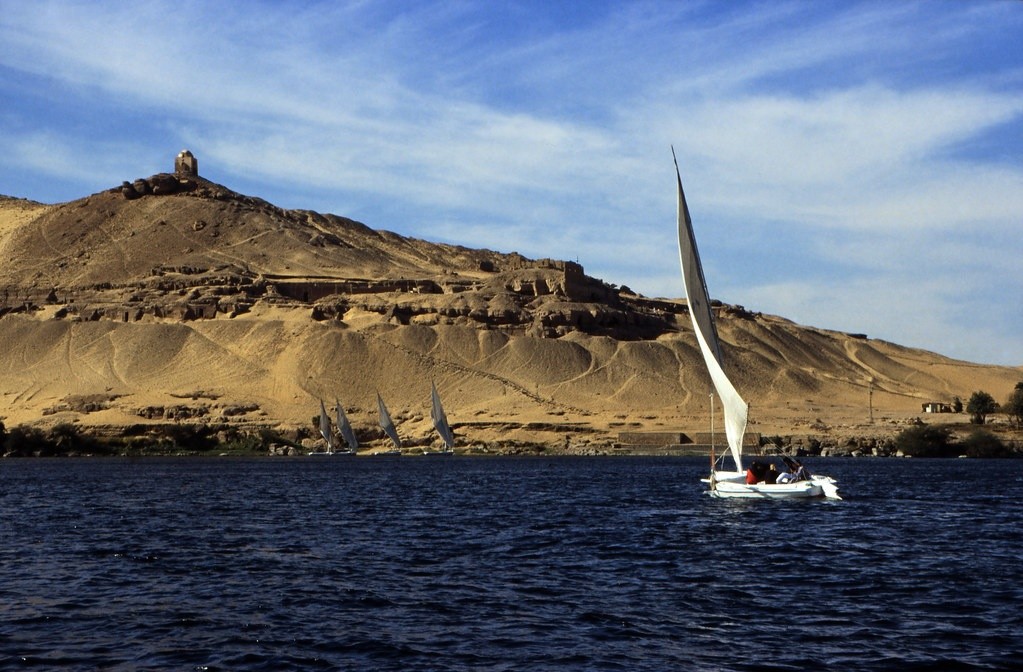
746 460 779 485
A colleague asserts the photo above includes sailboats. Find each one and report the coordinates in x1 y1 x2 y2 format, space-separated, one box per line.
669 145 843 500
369 389 404 455
333 396 360 457
422 379 457 457
302 398 336 456
